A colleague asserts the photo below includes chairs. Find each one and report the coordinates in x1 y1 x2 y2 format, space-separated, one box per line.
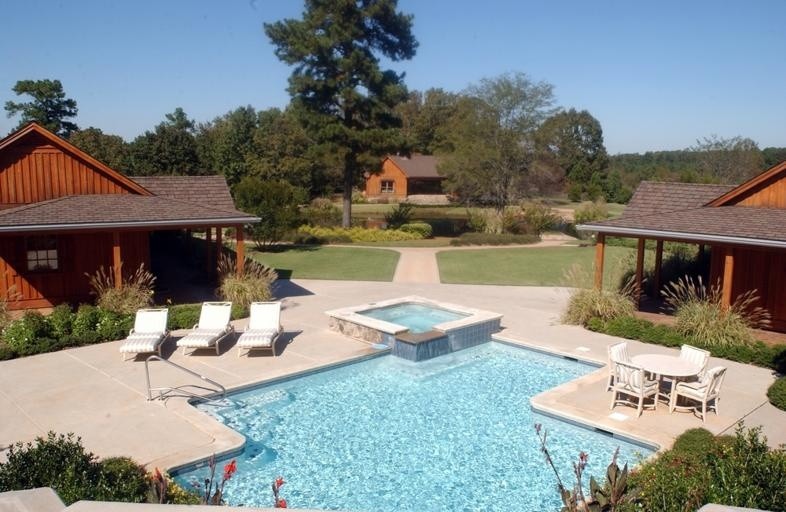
602 339 729 423
174 301 237 357
236 301 285 359
118 307 171 363
